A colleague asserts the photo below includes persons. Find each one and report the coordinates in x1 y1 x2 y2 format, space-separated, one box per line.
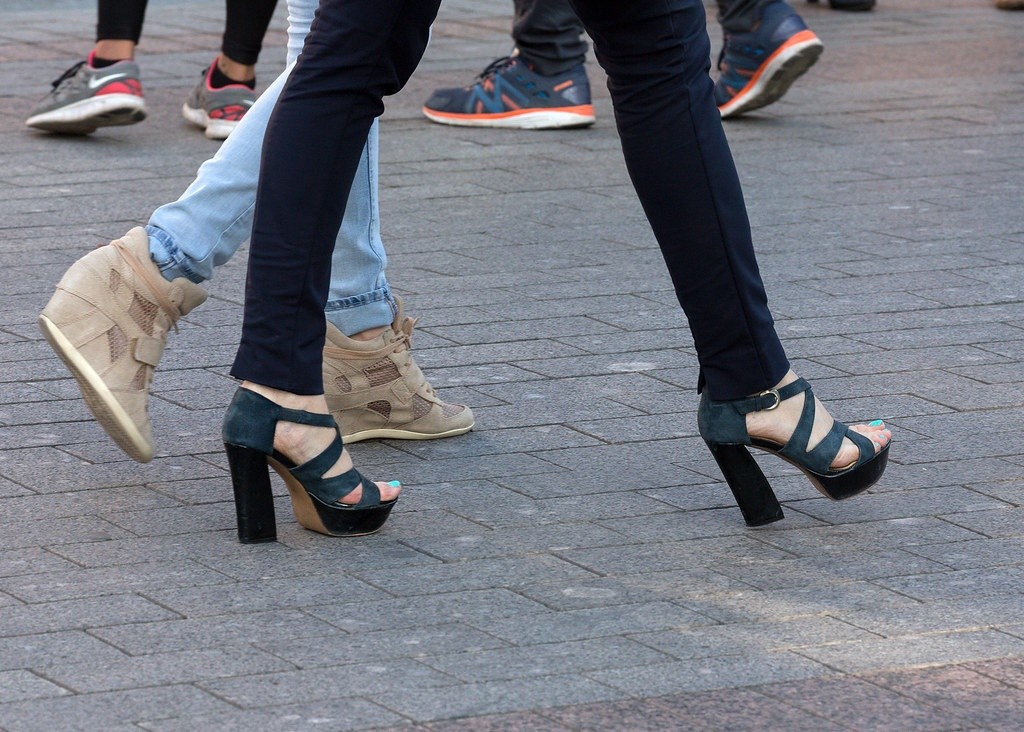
26 0 280 140
39 1 477 464
422 0 825 131
220 0 893 544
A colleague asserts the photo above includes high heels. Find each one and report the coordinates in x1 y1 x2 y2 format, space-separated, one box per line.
322 296 475 444
223 386 397 545
38 226 208 463
696 376 891 527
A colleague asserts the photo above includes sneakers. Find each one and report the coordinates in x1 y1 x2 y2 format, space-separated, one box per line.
181 55 256 139
421 48 595 130
27 50 148 133
714 1 824 117
